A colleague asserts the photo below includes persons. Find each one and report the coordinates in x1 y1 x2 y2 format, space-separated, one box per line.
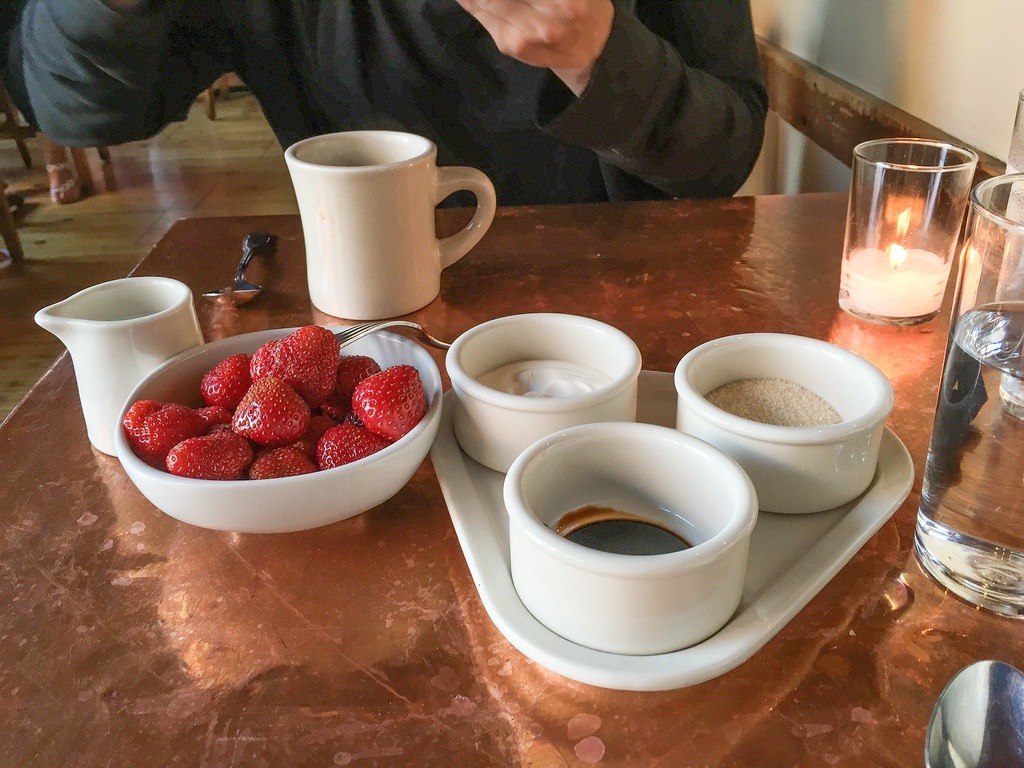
0 0 768 210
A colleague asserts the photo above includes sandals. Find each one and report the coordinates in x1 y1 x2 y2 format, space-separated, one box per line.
46 163 82 204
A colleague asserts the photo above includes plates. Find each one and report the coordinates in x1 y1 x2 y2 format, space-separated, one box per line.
429 367 916 691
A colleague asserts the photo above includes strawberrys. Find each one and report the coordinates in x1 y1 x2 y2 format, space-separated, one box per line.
123 325 427 480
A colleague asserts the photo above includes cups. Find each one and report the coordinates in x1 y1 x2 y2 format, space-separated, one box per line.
949 171 1024 332
1004 90 1024 176
913 172 1023 623
284 130 498 324
839 135 980 326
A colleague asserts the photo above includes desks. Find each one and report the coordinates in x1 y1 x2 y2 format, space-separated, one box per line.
0 189 1024 768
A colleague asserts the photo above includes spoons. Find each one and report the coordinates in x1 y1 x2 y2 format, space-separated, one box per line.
923 658 1024 768
202 230 273 307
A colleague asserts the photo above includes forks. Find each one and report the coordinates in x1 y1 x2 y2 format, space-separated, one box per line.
333 320 452 350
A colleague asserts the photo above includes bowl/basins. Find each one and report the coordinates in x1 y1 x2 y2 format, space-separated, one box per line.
444 310 643 475
112 323 445 533
673 331 894 514
502 421 759 656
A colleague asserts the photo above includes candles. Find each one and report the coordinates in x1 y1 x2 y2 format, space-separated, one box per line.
847 206 949 317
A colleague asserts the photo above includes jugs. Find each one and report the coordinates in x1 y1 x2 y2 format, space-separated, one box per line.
34 274 206 456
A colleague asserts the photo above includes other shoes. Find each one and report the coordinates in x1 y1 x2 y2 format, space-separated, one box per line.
213 85 253 99
8 194 24 216
